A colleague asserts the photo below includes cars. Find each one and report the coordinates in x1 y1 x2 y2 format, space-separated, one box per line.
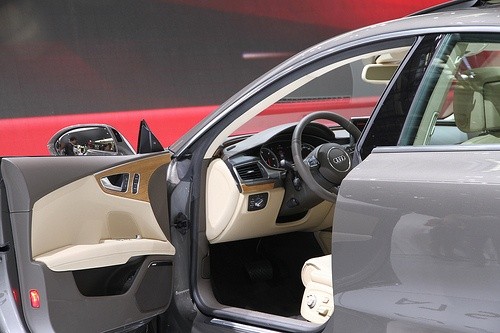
0 0 500 333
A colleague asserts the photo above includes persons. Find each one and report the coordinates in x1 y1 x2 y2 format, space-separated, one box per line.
56 140 115 154
66 137 77 156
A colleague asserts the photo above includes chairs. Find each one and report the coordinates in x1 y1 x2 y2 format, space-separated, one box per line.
298 66 500 327
